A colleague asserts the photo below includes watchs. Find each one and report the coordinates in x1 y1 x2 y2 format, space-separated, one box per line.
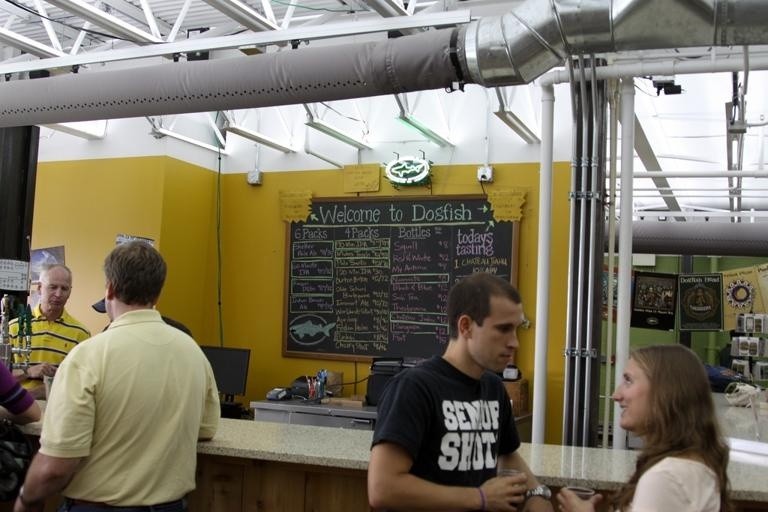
527 484 552 500
19 484 45 506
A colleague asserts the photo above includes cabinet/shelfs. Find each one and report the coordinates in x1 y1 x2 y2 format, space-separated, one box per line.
731 313 768 389
250 399 377 430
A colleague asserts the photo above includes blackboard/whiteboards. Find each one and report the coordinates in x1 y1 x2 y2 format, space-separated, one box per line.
282 193 521 372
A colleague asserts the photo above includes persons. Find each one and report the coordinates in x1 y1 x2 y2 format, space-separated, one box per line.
0 266 191 425
556 345 736 512
13 240 220 512
367 273 556 512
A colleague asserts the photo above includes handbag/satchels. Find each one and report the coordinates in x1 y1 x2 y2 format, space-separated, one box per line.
0 417 33 503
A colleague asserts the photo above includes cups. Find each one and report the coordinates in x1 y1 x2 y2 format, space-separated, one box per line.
498 468 526 512
562 486 595 512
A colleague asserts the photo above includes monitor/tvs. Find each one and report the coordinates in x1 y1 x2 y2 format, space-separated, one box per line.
199 345 252 396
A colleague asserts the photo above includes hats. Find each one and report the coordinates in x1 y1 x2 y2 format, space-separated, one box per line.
92 297 106 313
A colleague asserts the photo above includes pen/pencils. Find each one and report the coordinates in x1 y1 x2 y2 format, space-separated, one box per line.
305 369 328 399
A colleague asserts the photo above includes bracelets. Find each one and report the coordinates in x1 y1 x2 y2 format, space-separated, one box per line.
478 487 486 512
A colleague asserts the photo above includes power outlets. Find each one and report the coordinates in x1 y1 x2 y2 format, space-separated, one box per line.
478 167 493 183
